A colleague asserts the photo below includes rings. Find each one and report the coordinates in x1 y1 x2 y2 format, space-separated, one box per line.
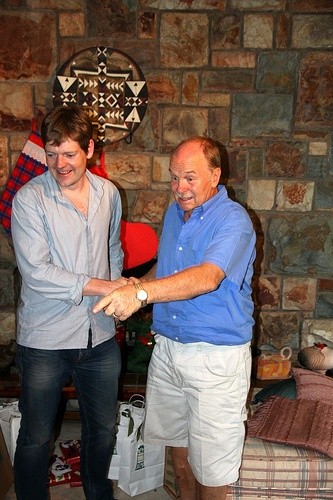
113 313 120 319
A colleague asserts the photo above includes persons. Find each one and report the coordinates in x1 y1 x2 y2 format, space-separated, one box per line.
10 108 128 500
91 137 257 500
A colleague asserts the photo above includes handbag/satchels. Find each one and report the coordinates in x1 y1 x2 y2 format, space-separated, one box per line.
117 410 165 497
0 401 22 467
107 394 146 479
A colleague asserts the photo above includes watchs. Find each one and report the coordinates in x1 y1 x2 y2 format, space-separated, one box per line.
134 282 149 309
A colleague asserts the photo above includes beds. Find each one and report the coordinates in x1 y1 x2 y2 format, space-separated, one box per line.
225 365 333 500
